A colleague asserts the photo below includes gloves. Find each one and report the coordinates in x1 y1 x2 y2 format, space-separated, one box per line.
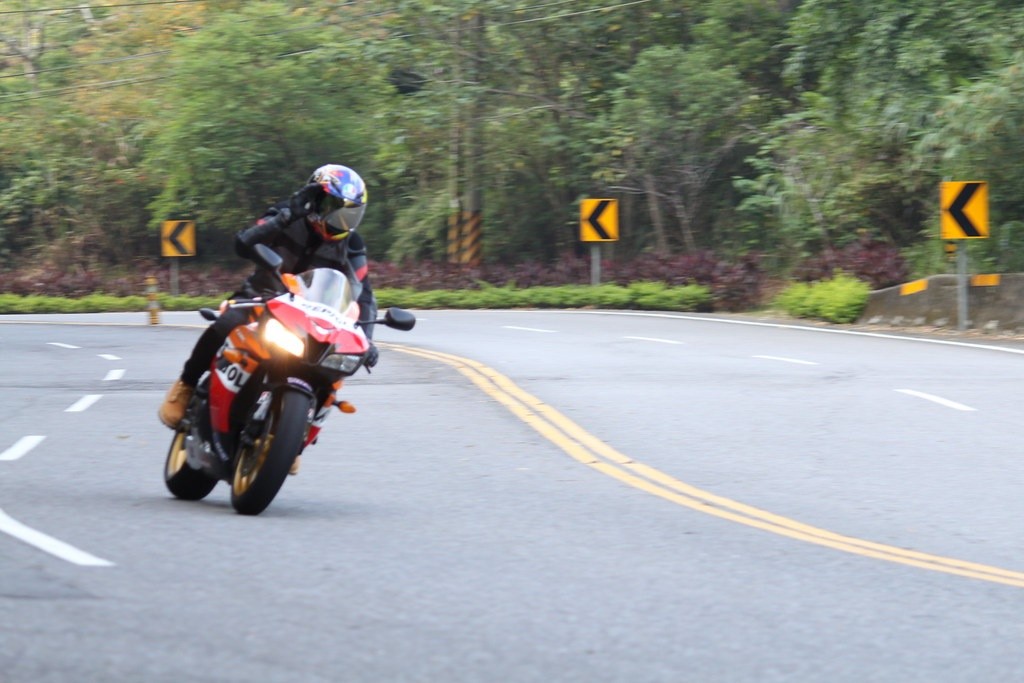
364 341 379 367
280 183 326 224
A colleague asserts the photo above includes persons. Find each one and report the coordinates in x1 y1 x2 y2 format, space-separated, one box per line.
157 162 379 476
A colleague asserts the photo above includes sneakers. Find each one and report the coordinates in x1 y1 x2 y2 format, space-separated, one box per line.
288 456 301 475
159 378 193 429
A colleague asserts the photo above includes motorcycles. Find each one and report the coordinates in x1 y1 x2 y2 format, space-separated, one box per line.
162 242 417 516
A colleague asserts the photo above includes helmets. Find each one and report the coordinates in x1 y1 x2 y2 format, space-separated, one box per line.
304 165 367 243
